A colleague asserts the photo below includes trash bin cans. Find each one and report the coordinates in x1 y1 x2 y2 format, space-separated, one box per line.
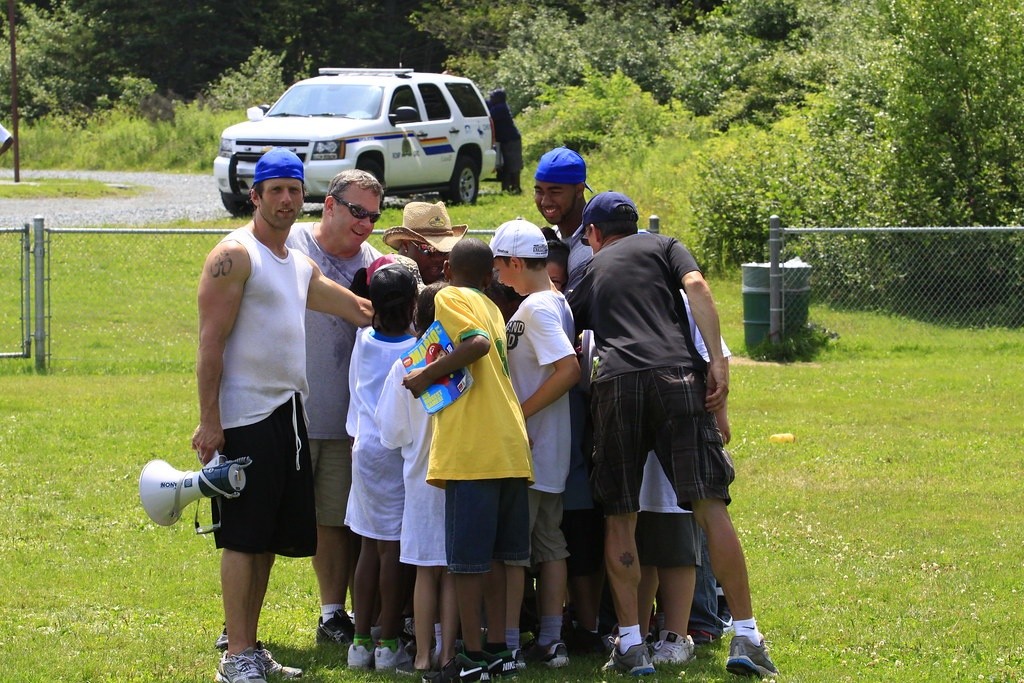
741 265 813 349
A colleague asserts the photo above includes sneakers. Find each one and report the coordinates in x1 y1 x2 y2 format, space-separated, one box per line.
421 653 492 683
486 650 520 681
253 647 303 678
374 637 413 670
510 648 527 669
602 636 655 675
317 599 733 644
522 638 569 667
724 633 778 677
214 647 267 683
651 630 696 666
347 644 375 668
216 621 229 649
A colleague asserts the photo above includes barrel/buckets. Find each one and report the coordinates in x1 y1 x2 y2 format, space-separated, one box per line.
740 262 811 356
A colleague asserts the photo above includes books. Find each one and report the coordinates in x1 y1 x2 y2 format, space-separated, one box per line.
400 320 476 416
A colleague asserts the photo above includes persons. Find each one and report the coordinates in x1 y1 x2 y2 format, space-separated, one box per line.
345 269 421 672
488 216 581 669
401 238 536 683
191 149 374 683
567 192 778 678
215 168 385 650
377 282 458 677
366 148 733 650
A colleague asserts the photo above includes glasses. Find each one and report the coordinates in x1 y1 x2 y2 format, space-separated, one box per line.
581 223 592 246
409 239 450 255
330 193 381 223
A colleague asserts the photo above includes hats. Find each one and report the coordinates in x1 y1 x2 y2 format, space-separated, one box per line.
254 145 305 184
488 217 548 258
571 190 640 239
382 201 468 253
534 146 594 193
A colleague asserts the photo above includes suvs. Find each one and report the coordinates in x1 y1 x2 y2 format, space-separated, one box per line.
213 68 499 218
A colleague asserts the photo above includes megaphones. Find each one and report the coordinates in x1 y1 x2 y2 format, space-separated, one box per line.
138 448 247 526
